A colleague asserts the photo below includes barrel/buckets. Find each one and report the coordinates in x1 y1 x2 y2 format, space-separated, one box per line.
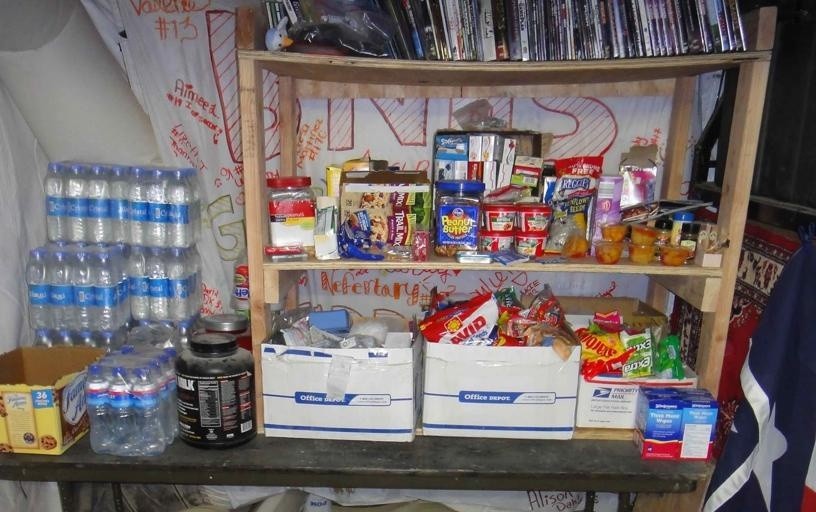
174 314 255 448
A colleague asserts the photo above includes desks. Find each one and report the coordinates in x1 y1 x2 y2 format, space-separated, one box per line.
0 410 699 512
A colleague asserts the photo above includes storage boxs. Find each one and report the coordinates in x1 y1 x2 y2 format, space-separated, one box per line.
551 288 673 336
421 299 585 441
258 309 424 446
574 347 701 434
0 343 105 458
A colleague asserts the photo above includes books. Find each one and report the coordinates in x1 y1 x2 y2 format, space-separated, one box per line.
265 1 748 63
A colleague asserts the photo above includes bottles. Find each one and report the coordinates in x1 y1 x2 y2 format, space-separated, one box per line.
25 242 199 344
41 158 203 249
87 344 179 456
233 249 248 309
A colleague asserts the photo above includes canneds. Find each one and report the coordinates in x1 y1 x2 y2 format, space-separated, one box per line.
434 179 485 256
655 209 701 265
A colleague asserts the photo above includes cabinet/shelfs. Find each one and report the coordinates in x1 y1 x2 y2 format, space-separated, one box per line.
233 0 782 438
